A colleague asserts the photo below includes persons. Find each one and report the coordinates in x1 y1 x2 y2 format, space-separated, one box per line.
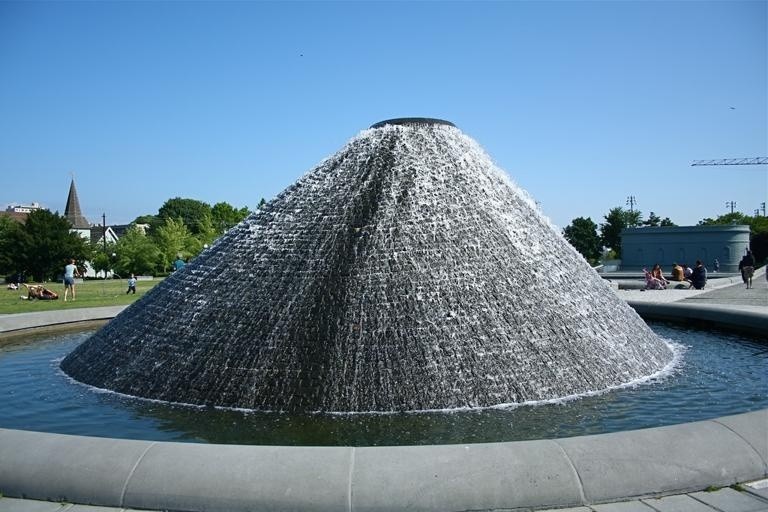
739 250 755 288
7 258 80 301
174 254 185 270
127 272 137 295
642 260 707 290
